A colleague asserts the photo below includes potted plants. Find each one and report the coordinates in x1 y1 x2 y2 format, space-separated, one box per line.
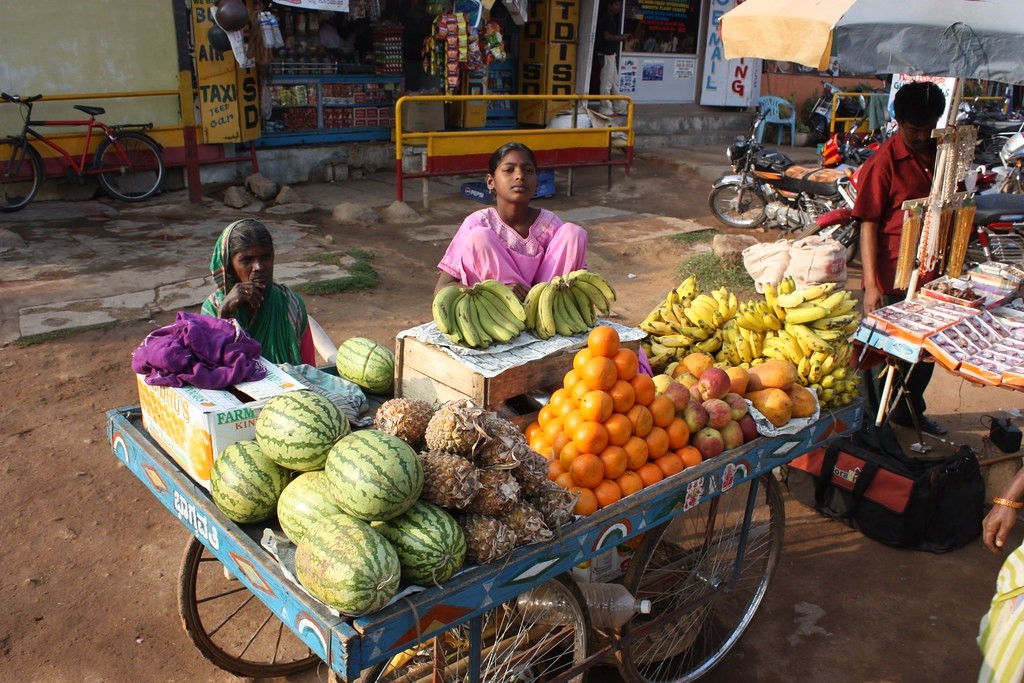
768 92 813 146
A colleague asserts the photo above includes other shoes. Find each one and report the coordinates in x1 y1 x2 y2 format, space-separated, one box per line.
890 412 947 435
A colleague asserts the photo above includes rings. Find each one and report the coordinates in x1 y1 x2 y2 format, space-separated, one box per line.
244 289 248 294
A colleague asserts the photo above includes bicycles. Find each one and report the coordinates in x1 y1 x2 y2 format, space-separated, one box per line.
0 90 167 213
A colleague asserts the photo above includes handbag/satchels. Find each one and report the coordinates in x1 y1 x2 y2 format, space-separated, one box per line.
785 441 989 554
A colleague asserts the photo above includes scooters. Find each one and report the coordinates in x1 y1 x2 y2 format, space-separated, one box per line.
956 97 1024 197
806 80 862 146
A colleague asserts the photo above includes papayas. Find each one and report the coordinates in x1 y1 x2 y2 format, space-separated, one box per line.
671 353 818 427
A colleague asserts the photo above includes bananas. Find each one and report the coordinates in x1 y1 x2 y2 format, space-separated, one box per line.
432 279 527 348
637 274 864 409
524 268 616 339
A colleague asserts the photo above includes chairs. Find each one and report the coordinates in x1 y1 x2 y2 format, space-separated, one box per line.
757 94 797 148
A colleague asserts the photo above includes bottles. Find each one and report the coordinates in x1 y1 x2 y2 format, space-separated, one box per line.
257 12 285 49
518 580 650 629
279 48 333 74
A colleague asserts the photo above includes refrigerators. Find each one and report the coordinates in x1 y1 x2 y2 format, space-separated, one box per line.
618 54 699 104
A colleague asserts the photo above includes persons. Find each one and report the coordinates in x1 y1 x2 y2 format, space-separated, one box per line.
975 466 1024 683
433 142 587 302
850 82 948 435
201 219 338 367
597 0 628 115
320 13 354 55
624 31 693 53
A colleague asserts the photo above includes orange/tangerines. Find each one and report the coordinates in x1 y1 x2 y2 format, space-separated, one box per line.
525 326 703 515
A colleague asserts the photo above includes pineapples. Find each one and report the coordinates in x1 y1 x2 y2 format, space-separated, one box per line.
375 398 575 563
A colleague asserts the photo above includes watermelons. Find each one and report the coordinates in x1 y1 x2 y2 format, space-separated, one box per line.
335 335 395 394
210 388 468 618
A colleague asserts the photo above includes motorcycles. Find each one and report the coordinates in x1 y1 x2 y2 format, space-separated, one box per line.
796 163 1024 267
818 113 900 169
706 109 855 229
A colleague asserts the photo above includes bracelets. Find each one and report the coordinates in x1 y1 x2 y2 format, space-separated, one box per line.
993 496 1024 508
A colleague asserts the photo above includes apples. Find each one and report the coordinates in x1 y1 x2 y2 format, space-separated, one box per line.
649 360 759 460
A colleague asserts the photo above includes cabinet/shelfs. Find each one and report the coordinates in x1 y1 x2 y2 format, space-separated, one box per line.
486 60 518 128
237 74 404 149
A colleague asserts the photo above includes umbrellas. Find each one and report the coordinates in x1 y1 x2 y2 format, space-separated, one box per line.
717 0 1024 87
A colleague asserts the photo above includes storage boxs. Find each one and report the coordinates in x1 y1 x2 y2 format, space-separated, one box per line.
138 353 311 493
868 259 1024 387
402 92 446 131
